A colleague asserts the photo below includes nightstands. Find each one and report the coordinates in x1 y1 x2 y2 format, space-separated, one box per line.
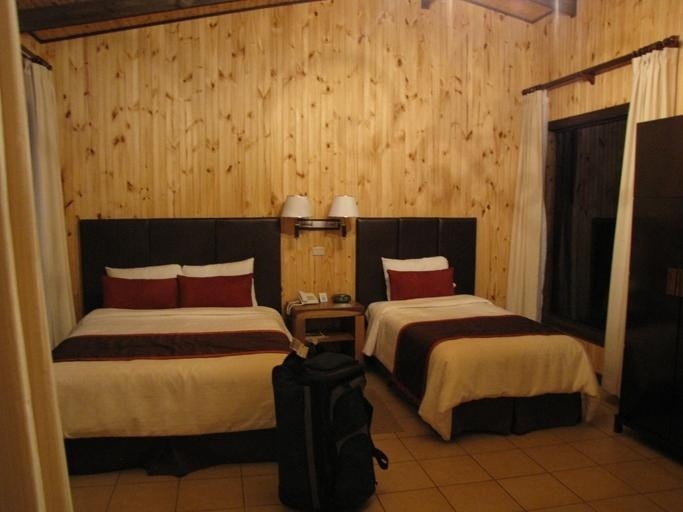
287 298 366 364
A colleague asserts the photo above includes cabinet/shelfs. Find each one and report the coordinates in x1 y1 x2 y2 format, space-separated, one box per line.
615 116 683 457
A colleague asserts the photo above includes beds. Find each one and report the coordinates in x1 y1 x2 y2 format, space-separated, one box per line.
51 216 293 471
354 215 586 442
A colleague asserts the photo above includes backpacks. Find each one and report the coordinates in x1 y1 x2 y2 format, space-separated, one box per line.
270 347 377 512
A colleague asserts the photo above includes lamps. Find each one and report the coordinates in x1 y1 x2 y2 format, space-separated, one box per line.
280 193 359 240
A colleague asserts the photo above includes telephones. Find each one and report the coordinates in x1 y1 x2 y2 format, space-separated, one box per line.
296 291 319 304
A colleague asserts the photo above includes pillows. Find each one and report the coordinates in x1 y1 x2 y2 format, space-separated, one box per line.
381 256 455 301
98 257 258 310
